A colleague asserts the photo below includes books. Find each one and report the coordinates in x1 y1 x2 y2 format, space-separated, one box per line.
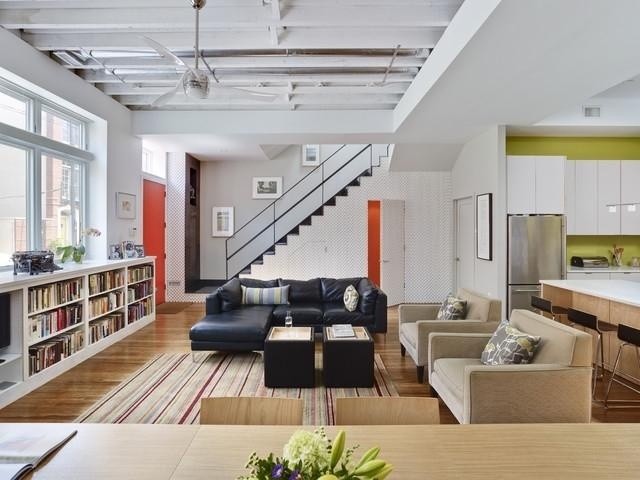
27 264 154 377
332 324 355 338
0 428 77 480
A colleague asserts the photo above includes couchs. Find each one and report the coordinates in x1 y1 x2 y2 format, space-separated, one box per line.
189 277 387 351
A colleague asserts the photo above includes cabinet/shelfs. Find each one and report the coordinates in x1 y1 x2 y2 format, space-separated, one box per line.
507 155 567 215
566 160 640 236
0 256 157 410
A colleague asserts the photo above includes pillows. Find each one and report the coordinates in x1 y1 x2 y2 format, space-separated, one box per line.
436 292 467 320
480 319 542 366
219 277 378 314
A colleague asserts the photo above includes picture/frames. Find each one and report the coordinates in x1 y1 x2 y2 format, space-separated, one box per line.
116 192 136 220
109 241 145 258
252 177 282 200
211 206 235 237
301 144 320 166
476 193 492 261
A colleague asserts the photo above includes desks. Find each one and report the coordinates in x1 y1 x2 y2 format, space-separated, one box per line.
1 422 639 479
539 279 639 385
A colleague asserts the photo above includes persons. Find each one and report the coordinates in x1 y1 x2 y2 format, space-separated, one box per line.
137 249 143 256
114 246 121 259
126 244 133 257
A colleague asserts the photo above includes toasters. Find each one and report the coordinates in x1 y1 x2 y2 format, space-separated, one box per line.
570 255 610 269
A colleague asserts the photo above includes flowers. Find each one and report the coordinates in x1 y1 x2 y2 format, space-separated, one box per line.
236 426 393 480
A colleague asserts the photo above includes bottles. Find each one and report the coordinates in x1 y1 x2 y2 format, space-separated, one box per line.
632 256 640 267
284 310 293 330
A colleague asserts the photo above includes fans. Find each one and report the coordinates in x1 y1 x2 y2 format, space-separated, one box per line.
129 1 276 107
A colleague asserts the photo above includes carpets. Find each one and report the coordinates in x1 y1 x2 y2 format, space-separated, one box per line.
73 352 400 424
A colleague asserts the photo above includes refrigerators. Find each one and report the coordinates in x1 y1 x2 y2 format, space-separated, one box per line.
507 214 567 320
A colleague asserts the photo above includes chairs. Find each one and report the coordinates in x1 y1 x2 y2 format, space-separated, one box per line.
398 289 501 383
530 295 640 409
428 309 594 424
200 396 441 425
